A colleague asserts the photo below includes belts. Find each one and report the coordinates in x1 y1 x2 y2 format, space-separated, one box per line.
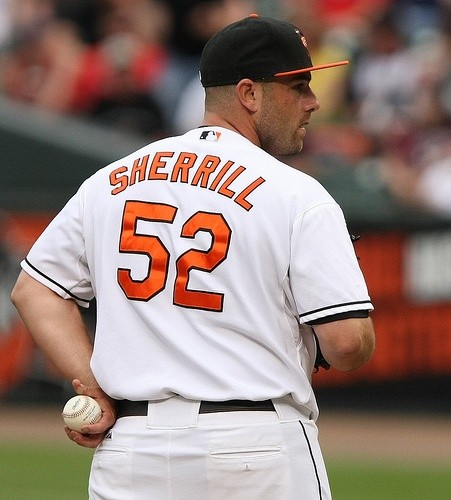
112 399 276 420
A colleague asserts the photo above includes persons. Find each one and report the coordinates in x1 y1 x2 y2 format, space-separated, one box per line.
11 14 375 499
0 0 450 260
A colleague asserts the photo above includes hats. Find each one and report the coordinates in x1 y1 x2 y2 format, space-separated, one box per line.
198 13 349 87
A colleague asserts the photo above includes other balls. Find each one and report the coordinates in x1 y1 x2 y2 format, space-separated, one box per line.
63 395 102 433
